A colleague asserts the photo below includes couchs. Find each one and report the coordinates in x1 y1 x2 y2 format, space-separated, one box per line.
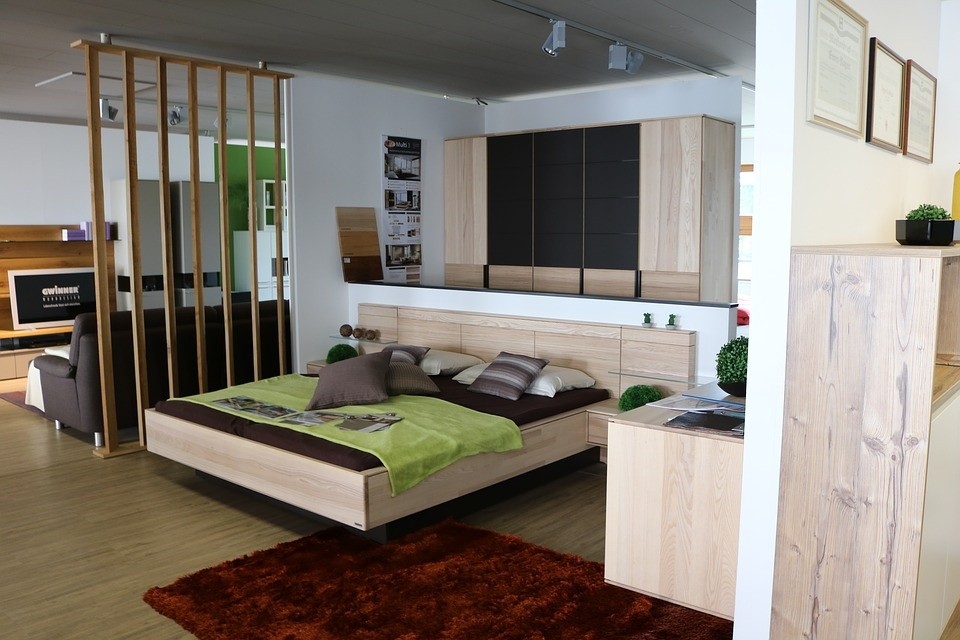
32 298 291 447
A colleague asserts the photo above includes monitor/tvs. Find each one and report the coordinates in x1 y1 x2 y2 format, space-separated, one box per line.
7 267 96 330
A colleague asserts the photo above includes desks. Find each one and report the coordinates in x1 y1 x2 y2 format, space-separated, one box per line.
604 381 744 620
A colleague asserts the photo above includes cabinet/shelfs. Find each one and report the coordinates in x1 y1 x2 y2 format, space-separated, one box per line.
769 244 959 640
0 223 116 380
440 113 737 303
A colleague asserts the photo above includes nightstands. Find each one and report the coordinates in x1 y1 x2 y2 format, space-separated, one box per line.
585 399 622 464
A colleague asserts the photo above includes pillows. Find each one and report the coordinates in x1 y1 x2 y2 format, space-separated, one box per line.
305 340 599 412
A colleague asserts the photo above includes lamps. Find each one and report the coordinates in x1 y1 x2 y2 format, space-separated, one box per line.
626 50 645 74
542 31 559 57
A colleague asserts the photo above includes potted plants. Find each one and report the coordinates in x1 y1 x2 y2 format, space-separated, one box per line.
665 314 678 330
716 335 747 397
642 313 652 328
896 203 955 245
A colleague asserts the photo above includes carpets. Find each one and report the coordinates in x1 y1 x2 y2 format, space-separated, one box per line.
0 391 46 418
142 513 733 640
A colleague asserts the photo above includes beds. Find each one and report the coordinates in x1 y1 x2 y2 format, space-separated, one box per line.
143 301 698 534
